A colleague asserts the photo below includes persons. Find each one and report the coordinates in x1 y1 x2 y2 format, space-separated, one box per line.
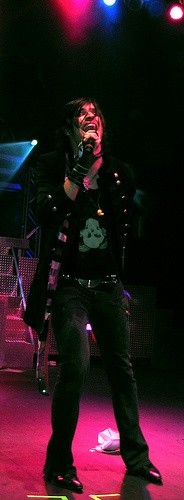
29 99 164 492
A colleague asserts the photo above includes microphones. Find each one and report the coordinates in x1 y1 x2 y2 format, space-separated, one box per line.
85 131 99 152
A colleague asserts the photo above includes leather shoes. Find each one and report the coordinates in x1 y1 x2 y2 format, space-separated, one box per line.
126 460 162 481
44 463 83 490
